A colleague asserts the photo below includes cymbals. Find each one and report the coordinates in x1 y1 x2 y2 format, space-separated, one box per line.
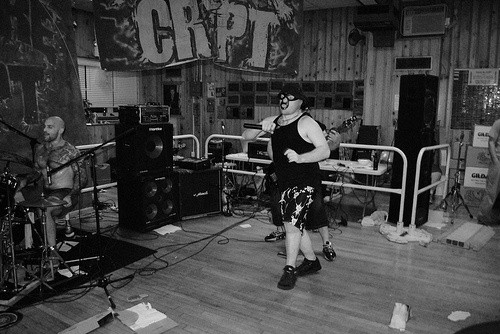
0 161 33 175
19 198 69 208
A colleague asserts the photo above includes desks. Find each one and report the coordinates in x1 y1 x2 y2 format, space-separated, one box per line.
225 152 392 218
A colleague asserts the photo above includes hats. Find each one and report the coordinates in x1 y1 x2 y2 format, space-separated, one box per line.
277 83 308 110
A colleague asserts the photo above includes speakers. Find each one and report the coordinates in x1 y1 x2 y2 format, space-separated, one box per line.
114 124 224 232
388 74 439 227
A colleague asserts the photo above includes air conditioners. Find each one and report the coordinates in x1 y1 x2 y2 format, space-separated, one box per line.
401 5 444 37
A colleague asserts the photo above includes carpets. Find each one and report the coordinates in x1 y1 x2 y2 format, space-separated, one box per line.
0 221 158 311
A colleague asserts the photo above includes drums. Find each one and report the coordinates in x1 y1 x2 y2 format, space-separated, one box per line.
0 172 19 218
0 218 49 267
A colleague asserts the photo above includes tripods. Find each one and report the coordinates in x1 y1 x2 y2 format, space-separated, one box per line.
0 160 135 309
433 131 474 224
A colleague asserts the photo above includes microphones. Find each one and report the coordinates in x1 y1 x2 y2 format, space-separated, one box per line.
243 123 276 131
46 163 51 185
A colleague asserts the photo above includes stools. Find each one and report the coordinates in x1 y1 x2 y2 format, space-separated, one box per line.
56 201 80 238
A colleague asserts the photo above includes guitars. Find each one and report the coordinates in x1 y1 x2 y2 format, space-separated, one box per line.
325 116 365 141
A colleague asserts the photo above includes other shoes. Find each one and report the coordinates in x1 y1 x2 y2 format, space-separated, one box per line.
42 251 59 269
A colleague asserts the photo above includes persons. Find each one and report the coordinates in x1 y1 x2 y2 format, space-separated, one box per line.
243 83 330 291
477 119 500 226
14 116 87 246
265 119 341 261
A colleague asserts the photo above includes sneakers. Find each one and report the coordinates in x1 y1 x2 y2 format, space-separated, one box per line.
323 241 336 260
265 231 286 241
278 265 296 289
296 257 322 276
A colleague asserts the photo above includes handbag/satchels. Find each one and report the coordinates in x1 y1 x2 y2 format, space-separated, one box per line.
362 211 388 226
